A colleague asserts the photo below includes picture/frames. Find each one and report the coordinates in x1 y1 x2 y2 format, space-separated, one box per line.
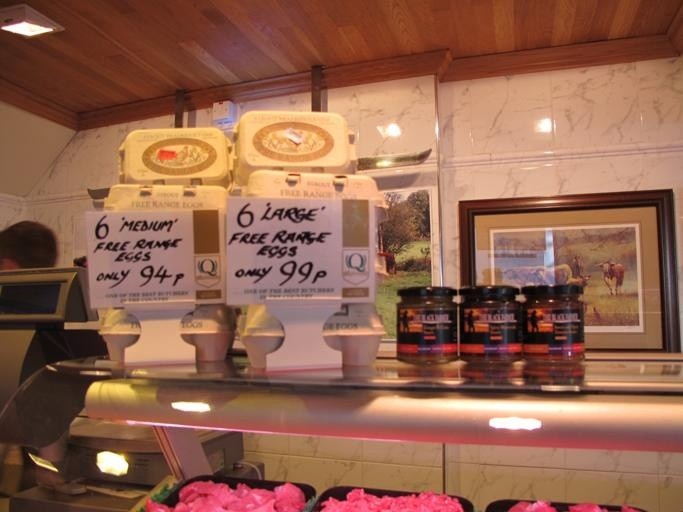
361 186 440 358
459 189 680 353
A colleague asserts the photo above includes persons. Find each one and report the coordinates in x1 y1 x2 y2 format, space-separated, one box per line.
570 255 583 278
0 221 58 270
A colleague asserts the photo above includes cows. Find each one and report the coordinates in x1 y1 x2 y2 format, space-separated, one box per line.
600 261 624 296
500 255 591 287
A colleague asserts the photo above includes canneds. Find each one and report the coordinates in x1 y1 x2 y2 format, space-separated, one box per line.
396 286 457 357
521 285 585 355
459 286 521 356
